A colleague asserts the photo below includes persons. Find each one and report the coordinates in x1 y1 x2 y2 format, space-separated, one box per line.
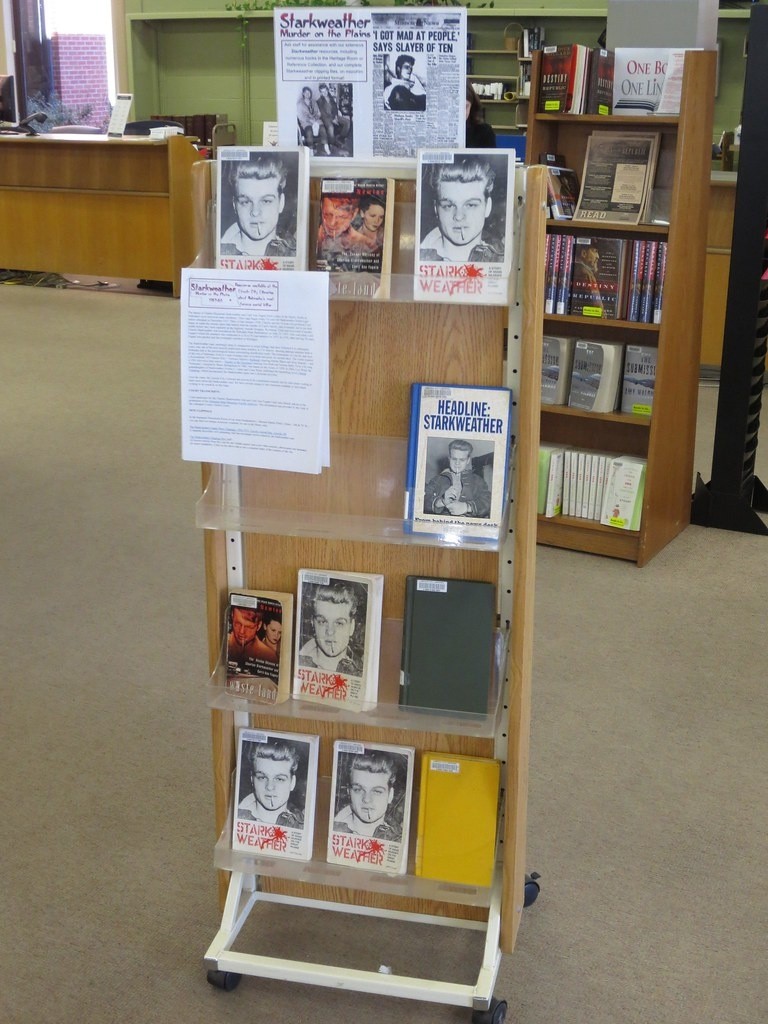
220 156 296 257
466 80 496 148
571 239 607 319
300 585 363 677
238 741 304 830
424 440 491 518
318 192 386 273
420 157 504 263
298 85 343 154
228 608 281 680
334 750 402 842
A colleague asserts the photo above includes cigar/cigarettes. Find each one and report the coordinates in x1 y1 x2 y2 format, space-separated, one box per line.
271 796 274 807
331 642 334 653
258 223 261 236
368 808 372 819
460 228 464 240
456 468 458 474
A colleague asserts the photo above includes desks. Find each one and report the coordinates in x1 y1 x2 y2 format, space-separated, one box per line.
0 130 210 297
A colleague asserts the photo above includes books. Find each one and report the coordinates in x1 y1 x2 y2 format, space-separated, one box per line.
215 145 311 272
315 176 396 300
520 27 546 96
537 43 667 532
405 383 512 542
415 148 517 303
225 590 293 705
292 569 495 720
231 726 500 888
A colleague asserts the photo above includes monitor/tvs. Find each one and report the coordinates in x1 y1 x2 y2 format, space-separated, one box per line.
0 75 19 135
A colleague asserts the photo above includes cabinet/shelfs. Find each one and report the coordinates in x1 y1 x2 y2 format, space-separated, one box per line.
465 14 531 150
178 121 549 1024
530 46 717 568
189 124 238 161
